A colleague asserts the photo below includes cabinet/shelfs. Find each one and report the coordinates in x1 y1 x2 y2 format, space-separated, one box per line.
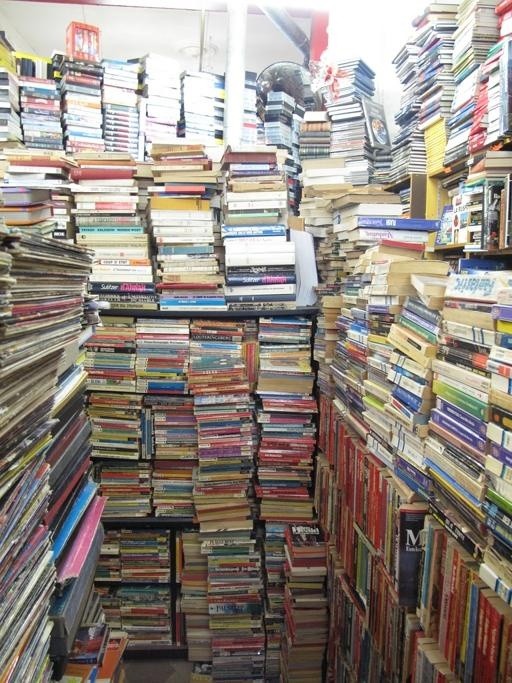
383 175 425 218
98 305 323 658
429 134 512 262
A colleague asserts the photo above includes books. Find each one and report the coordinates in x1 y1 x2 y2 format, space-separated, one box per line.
0 0 512 683
